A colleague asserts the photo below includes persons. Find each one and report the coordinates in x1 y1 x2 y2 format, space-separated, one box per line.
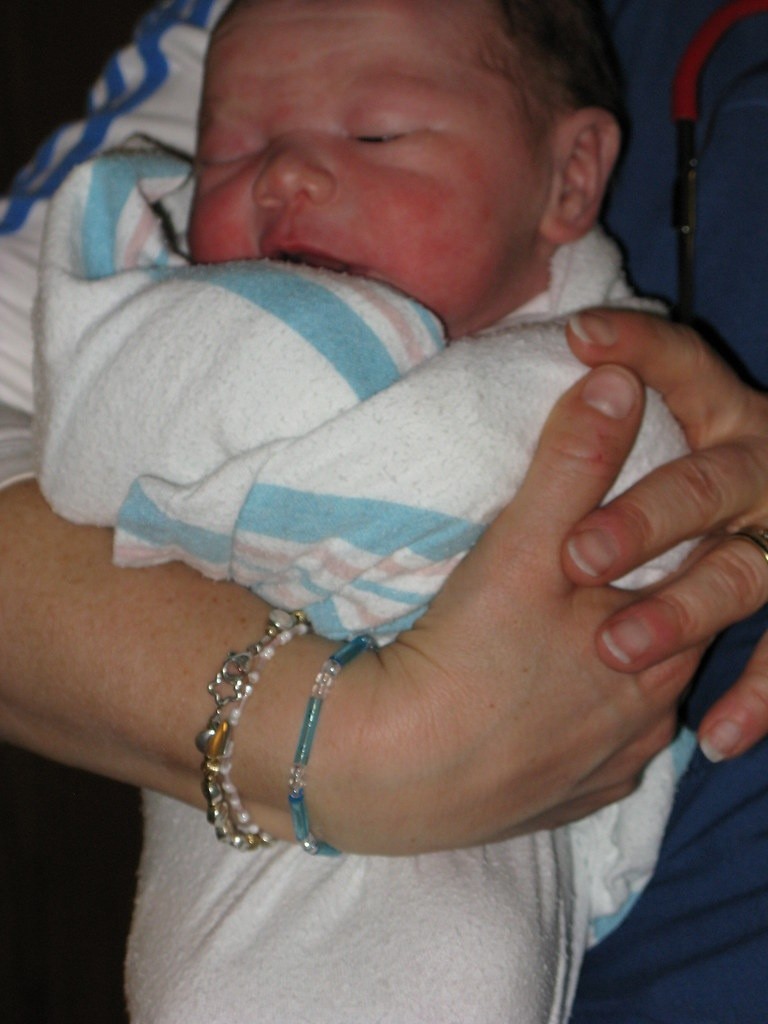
30 0 705 1024
0 0 768 1024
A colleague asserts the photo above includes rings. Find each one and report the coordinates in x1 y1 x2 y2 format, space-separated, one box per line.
730 525 768 562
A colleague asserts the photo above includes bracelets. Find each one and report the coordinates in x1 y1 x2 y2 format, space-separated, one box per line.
288 634 378 858
196 610 314 853
